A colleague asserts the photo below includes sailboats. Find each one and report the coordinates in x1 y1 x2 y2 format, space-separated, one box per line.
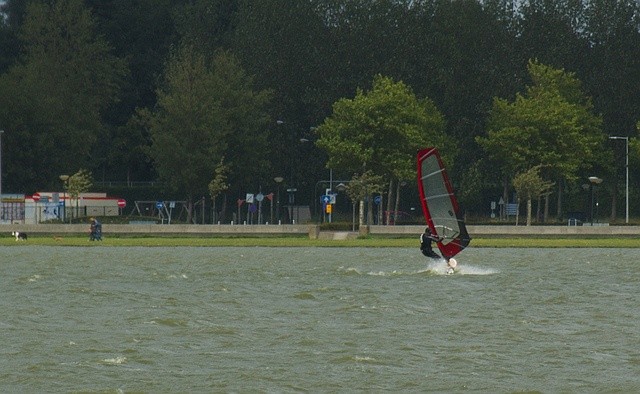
410 144 473 277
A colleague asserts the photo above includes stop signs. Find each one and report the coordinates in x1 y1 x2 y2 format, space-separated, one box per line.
118 199 126 207
33 194 40 201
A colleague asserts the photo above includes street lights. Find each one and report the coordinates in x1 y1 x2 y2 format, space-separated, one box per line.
608 137 628 225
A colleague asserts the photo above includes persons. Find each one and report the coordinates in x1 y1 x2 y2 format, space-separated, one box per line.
88 218 103 242
420 224 443 269
